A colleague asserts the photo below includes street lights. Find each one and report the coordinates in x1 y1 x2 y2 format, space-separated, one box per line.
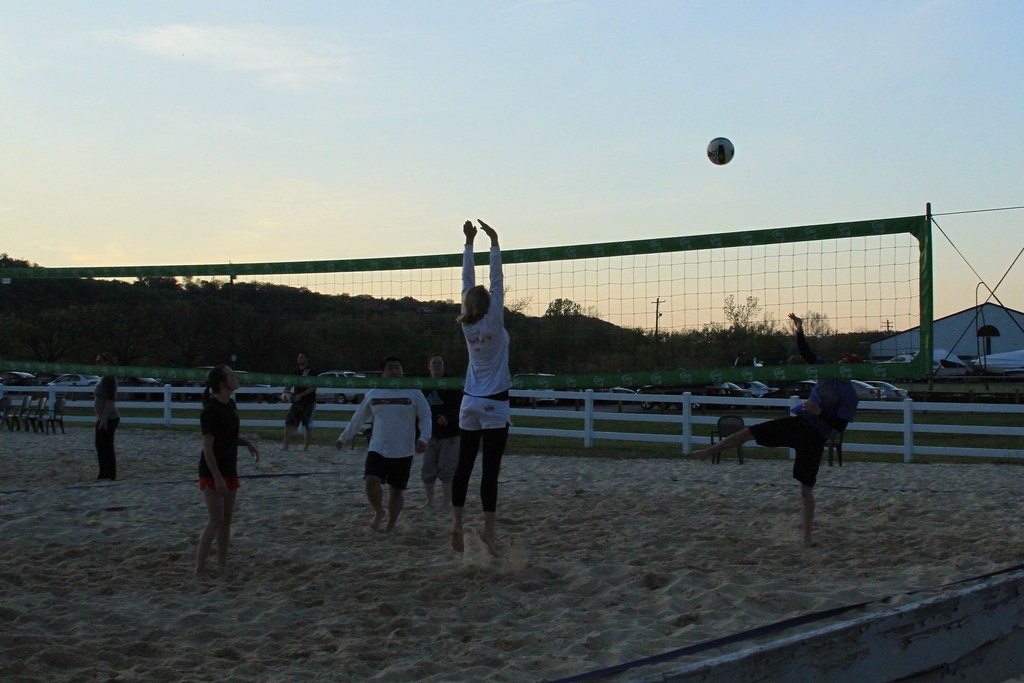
651 298 665 334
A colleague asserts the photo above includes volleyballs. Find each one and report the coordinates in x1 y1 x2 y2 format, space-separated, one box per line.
706 136 735 166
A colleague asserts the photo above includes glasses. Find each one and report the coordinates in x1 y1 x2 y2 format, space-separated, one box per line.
96 359 108 363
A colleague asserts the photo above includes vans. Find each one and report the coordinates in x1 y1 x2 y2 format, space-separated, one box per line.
510 373 555 404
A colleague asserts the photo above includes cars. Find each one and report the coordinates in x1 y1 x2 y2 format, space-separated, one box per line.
610 387 634 404
726 382 749 408
850 380 886 401
637 383 731 410
790 380 817 398
735 381 779 398
0 365 421 404
864 381 908 400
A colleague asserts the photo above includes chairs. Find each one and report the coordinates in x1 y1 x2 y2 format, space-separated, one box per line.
710 416 746 465
0 396 66 435
351 422 373 450
824 422 848 467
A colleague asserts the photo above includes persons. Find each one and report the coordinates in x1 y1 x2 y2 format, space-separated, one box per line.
193 365 260 581
684 313 862 547
279 352 318 452
337 356 432 532
0 377 7 399
416 353 462 506
93 352 119 481
451 219 509 558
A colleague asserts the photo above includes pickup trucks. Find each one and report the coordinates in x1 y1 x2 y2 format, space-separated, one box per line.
877 352 966 383
